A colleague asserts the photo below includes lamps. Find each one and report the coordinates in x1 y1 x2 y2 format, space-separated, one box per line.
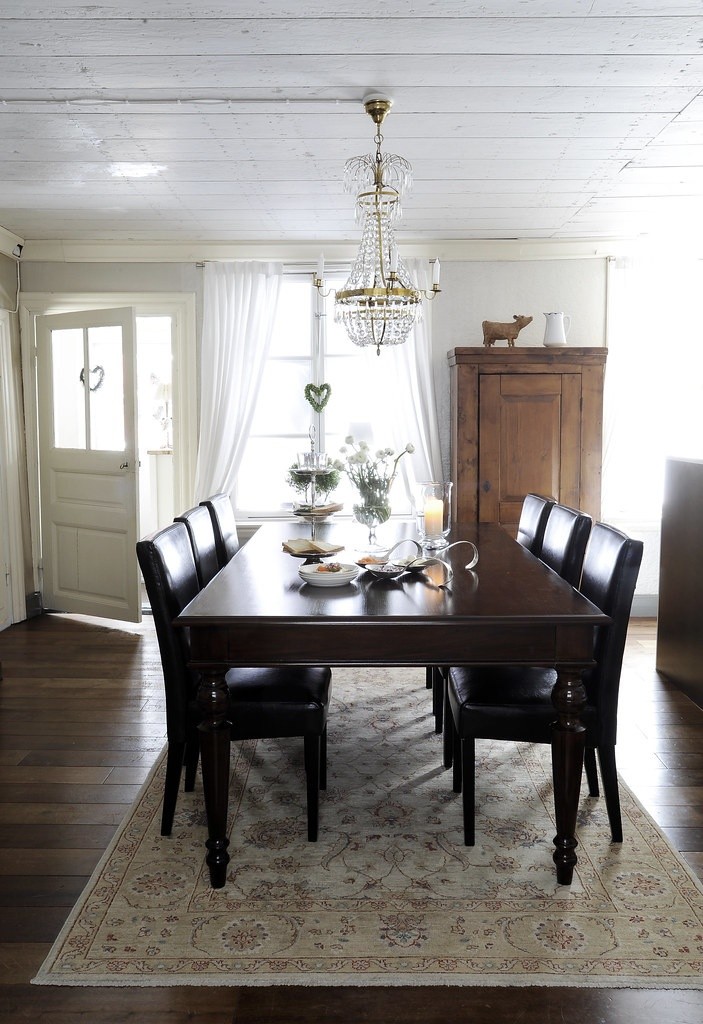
312 92 443 356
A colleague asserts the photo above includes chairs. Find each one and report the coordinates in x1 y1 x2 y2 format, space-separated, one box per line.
434 503 593 770
198 492 239 569
134 519 332 843
425 493 559 717
449 521 643 848
173 504 221 766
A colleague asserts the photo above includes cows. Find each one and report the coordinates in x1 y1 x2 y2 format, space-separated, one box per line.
481 314 534 348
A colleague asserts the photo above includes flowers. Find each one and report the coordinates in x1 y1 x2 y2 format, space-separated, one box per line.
332 433 415 478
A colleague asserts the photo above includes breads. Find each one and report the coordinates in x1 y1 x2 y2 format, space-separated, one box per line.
282 538 345 554
311 502 344 513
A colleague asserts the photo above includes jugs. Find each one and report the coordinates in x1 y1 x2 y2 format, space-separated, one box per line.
543 311 572 347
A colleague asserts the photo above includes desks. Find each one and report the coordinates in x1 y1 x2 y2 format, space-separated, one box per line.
174 520 612 890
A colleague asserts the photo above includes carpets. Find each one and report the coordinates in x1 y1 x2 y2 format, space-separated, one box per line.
29 668 703 991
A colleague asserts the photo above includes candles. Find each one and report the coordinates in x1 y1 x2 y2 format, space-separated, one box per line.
414 480 453 551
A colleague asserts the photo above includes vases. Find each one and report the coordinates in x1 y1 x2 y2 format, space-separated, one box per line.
349 475 396 554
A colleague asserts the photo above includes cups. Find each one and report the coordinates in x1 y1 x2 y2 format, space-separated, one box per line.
413 480 453 549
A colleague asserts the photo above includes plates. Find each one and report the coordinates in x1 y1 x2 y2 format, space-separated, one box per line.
298 562 360 587
355 539 481 589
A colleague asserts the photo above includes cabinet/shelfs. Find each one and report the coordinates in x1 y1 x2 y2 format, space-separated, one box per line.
446 347 609 539
